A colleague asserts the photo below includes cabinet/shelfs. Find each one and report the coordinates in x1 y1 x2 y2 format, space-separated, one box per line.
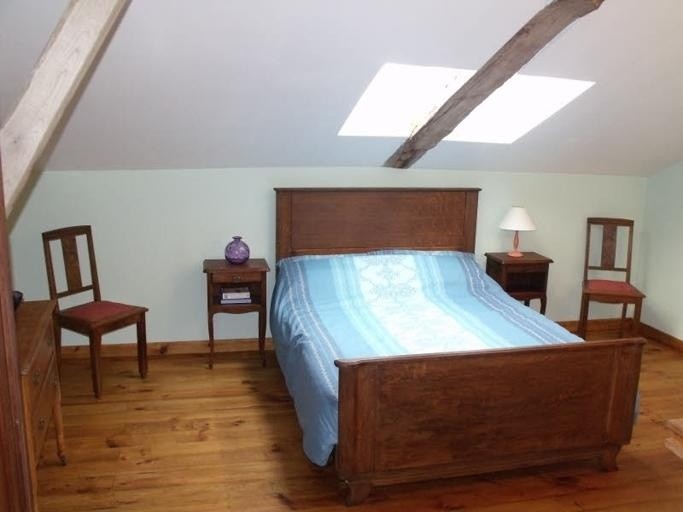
10 300 70 510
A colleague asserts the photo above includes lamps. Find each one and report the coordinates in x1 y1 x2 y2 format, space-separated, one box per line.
499 206 536 257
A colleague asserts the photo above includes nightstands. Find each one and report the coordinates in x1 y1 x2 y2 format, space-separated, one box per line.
203 258 270 368
483 251 553 314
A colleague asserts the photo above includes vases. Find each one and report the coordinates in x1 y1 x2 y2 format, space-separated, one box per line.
224 236 250 266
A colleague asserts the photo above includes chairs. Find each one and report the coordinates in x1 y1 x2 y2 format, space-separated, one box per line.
42 225 150 398
577 217 646 340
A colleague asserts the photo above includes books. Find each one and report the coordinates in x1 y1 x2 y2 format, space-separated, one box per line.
219 286 251 304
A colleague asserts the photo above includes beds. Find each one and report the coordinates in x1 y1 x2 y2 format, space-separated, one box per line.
268 187 645 507
